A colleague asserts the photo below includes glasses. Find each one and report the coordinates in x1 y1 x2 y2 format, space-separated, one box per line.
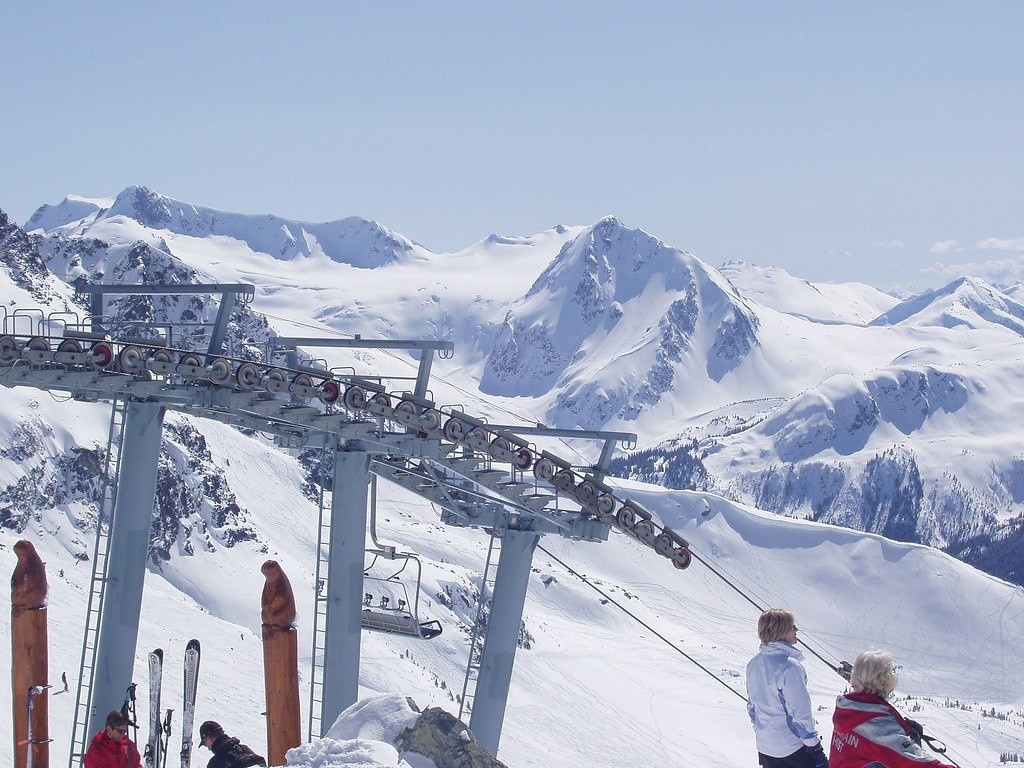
792 625 795 629
114 728 126 733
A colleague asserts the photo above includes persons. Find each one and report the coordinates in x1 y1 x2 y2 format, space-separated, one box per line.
84 711 144 768
745 608 830 768
826 649 960 768
198 721 266 768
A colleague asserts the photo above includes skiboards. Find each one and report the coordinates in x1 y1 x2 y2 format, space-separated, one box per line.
140 638 200 768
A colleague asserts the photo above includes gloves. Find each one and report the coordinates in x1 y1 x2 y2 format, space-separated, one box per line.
812 751 829 768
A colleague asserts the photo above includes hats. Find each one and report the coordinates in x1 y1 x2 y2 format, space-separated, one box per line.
198 721 222 748
